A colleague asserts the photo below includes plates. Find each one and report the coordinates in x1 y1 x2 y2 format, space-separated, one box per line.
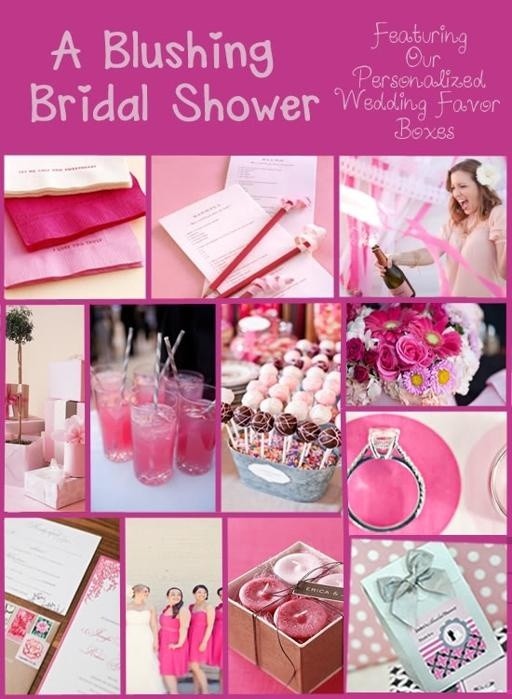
222 359 260 405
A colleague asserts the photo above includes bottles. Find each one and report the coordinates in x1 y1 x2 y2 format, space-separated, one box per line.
370 244 419 297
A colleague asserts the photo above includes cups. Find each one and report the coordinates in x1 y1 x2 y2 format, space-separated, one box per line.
87 358 217 486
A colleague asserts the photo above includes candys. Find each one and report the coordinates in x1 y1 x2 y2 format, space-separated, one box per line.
239 578 290 612
317 574 345 612
274 599 326 642
221 339 341 470
274 553 323 584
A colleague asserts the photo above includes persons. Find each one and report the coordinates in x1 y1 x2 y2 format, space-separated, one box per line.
187 584 215 695
208 587 224 694
374 157 508 297
158 588 192 694
126 584 169 694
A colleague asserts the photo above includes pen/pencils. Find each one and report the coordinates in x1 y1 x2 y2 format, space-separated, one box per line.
203 200 311 299
